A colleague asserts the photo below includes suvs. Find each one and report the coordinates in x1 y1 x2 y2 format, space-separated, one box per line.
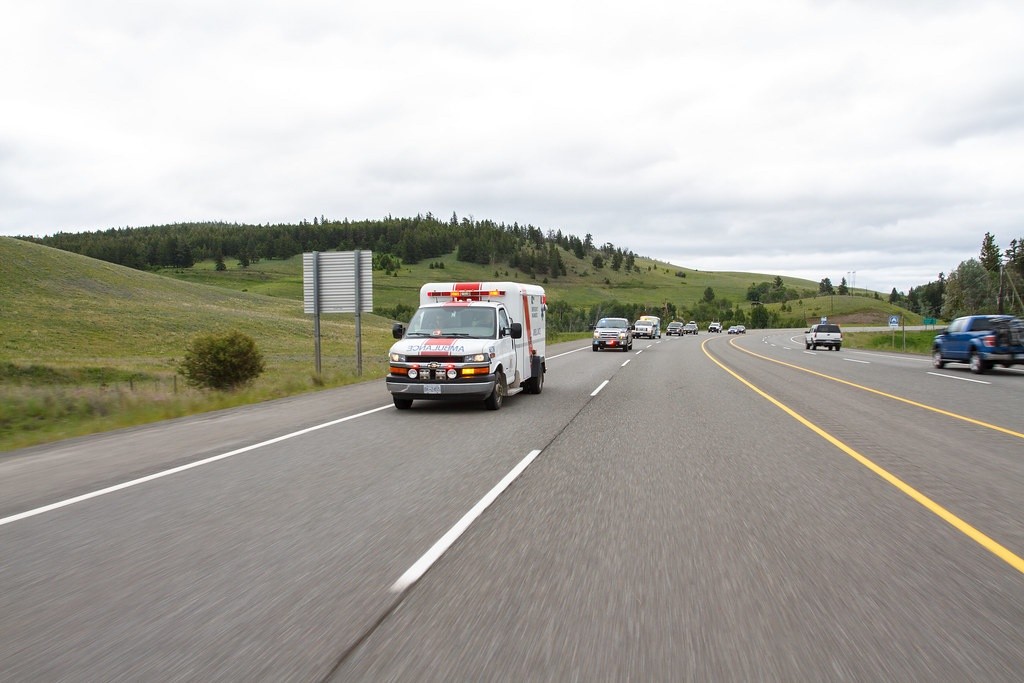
683 321 698 335
805 324 843 351
666 321 684 336
591 317 633 352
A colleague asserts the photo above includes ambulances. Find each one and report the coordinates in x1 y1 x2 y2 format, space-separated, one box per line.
385 280 549 411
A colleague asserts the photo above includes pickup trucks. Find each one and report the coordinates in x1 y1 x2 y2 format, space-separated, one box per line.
932 315 1024 374
631 320 657 339
707 322 723 333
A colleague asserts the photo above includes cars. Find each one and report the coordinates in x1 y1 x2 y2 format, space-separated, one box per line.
737 325 746 333
728 326 739 334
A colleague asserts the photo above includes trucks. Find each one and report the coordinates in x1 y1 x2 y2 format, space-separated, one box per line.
640 316 661 339
